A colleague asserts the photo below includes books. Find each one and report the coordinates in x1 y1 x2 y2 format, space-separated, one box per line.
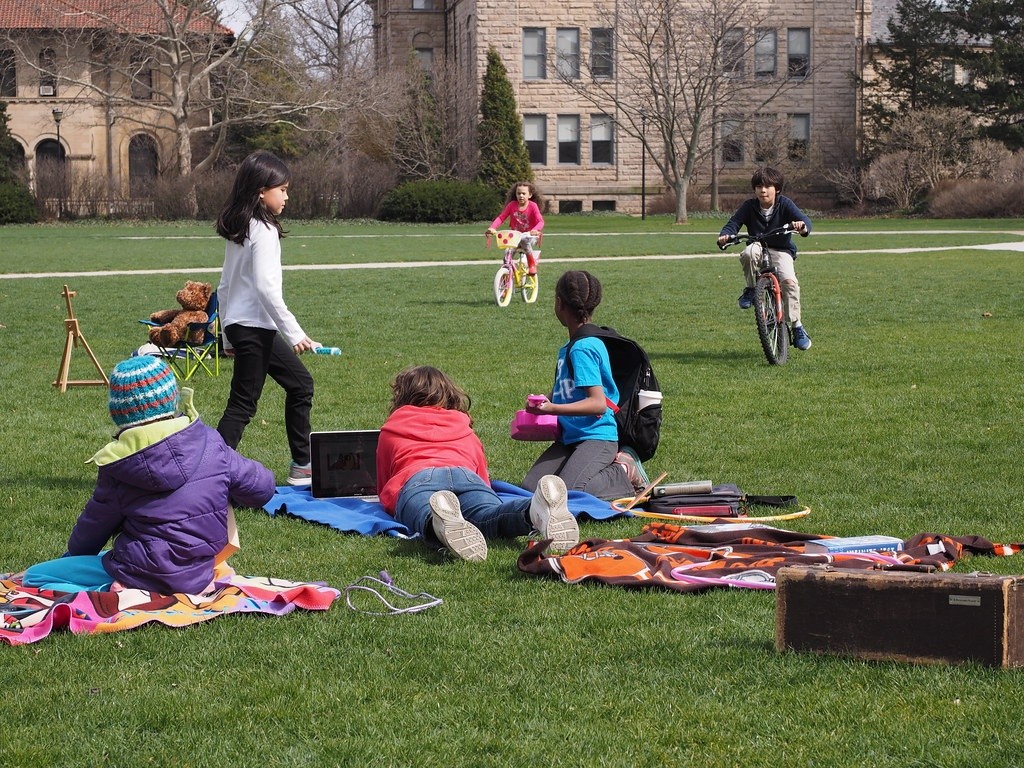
805 534 906 553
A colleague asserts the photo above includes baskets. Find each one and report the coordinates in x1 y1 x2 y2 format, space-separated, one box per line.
496 229 524 250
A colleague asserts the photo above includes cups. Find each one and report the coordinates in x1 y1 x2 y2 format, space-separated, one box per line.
638 390 663 411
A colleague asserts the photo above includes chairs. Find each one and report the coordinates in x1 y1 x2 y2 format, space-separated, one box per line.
136 292 219 381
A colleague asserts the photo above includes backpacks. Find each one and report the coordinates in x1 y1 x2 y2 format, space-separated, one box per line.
566 324 664 462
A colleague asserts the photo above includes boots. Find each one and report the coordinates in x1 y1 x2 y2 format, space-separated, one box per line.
526 253 538 275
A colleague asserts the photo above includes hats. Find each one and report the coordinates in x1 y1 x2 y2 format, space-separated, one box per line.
108 356 180 429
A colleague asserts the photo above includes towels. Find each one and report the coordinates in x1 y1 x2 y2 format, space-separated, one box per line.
261 479 636 539
1 565 341 650
514 517 1024 594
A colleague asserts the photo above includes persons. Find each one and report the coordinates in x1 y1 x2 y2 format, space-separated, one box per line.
485 182 544 298
22 354 274 594
716 167 814 352
377 367 580 565
518 269 651 501
215 150 323 486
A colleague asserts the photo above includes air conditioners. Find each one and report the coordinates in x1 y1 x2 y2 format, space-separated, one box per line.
40 86 54 96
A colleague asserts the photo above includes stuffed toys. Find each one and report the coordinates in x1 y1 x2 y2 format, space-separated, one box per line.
148 279 210 346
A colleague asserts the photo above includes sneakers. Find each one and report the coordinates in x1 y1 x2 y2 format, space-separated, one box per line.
430 489 488 563
738 286 756 309
615 444 651 495
791 324 811 351
285 461 312 486
528 474 580 556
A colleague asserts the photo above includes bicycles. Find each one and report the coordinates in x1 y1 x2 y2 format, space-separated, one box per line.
485 230 539 308
716 222 804 368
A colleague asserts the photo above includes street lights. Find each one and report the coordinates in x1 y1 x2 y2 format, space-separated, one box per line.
638 104 649 221
52 105 64 220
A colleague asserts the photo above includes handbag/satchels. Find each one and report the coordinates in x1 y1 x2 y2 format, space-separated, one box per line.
649 484 798 516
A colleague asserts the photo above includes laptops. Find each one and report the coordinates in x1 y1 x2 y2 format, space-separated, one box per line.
309 430 384 502
